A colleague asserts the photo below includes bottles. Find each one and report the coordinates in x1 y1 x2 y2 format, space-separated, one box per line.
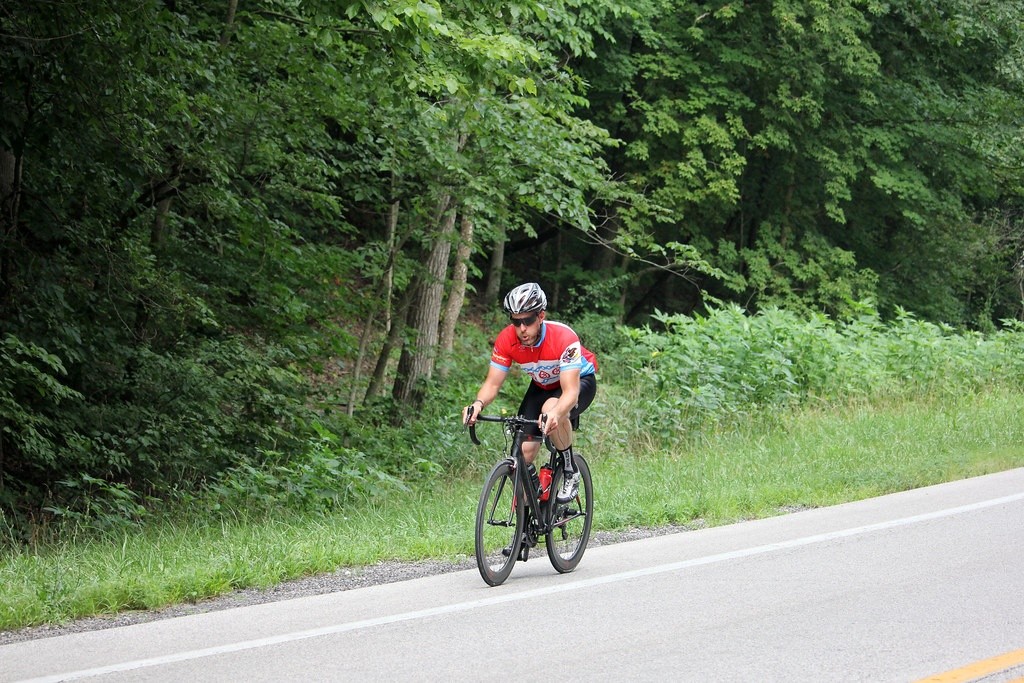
539 463 553 500
527 463 543 498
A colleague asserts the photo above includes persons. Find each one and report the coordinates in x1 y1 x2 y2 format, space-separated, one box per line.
462 283 599 557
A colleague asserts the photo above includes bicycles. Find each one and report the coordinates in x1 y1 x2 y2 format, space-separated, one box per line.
462 406 594 587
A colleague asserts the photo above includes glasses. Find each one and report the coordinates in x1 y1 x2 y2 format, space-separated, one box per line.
508 312 538 328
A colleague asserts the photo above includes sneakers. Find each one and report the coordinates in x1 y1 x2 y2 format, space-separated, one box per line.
557 462 581 503
502 533 528 560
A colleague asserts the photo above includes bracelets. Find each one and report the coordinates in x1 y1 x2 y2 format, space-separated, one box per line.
475 400 484 412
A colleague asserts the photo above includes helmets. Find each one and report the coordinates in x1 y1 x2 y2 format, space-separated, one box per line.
503 284 547 315
